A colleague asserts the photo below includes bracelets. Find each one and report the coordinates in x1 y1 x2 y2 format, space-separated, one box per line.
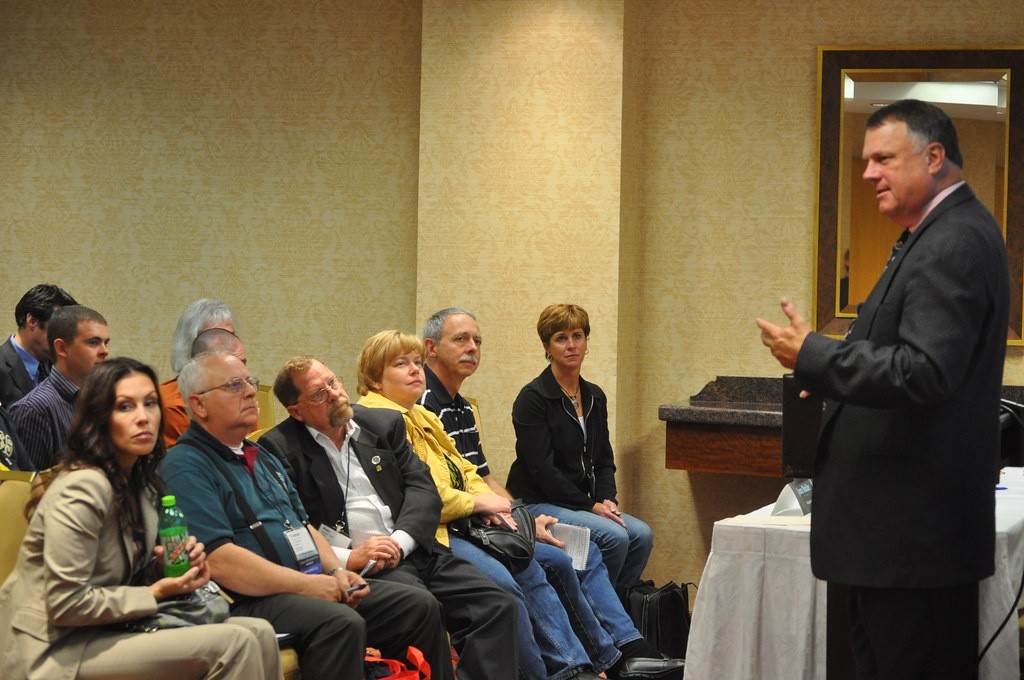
326 567 343 576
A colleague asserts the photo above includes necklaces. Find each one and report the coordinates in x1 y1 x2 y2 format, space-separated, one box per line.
560 386 580 410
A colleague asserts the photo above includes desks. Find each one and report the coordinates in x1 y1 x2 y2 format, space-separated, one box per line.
682 467 1024 680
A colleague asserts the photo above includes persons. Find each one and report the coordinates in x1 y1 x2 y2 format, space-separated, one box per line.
755 98 1011 680
356 330 594 680
7 305 111 470
155 351 455 680
0 405 45 496
505 303 654 604
257 355 520 680
415 307 685 680
159 298 260 446
0 357 284 680
0 281 79 411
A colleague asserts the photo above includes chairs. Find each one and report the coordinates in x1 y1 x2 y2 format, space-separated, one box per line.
0 382 302 680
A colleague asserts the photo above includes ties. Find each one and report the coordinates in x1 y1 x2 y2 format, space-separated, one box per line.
841 225 914 340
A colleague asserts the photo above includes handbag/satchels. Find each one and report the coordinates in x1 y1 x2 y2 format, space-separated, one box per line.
105 563 235 632
613 575 690 659
448 497 536 575
363 644 431 680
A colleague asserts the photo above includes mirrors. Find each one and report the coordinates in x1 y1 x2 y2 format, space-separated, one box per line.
813 42 1024 347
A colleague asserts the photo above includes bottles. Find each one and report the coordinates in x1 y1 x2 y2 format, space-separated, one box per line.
160 495 190 577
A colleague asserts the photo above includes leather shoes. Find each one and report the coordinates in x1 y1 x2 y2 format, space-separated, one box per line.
617 653 685 680
569 670 607 680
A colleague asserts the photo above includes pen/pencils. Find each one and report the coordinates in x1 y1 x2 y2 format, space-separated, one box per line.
346 582 367 595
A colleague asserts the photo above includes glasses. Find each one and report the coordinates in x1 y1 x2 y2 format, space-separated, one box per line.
196 377 261 395
287 375 342 406
